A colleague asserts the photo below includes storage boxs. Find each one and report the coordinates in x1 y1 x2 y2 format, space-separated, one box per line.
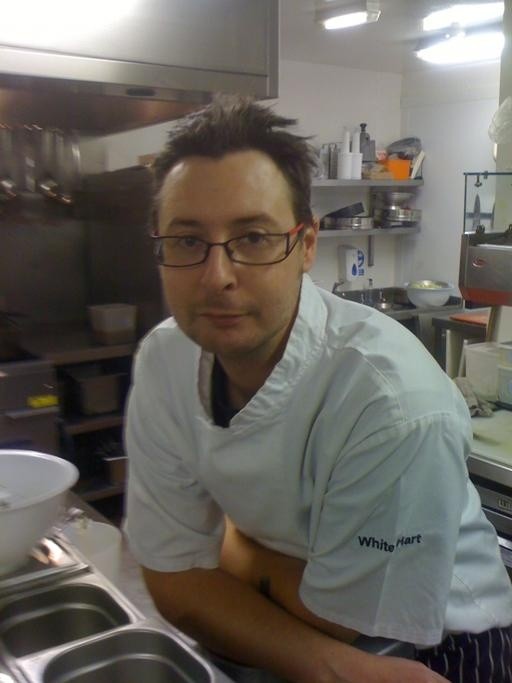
77 372 127 415
102 456 128 485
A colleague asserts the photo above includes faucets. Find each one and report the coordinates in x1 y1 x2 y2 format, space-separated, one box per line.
368 279 374 307
331 281 347 298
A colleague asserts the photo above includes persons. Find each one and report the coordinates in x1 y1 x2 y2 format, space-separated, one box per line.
120 91 511 682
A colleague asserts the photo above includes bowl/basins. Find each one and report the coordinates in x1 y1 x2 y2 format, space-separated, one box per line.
0 448 80 578
403 281 454 307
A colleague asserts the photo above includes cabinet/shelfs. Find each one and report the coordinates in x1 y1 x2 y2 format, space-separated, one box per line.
0 336 138 503
311 177 425 238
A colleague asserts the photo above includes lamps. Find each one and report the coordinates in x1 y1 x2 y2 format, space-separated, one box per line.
314 0 382 31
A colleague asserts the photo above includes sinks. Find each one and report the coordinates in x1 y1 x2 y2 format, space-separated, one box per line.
360 300 416 313
9 616 234 683
1 569 145 665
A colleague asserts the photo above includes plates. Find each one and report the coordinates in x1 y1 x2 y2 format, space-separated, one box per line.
323 201 422 231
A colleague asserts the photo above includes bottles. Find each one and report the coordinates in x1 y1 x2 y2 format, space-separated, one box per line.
337 123 370 180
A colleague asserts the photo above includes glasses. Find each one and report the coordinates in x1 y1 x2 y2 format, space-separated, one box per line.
150 223 305 268
